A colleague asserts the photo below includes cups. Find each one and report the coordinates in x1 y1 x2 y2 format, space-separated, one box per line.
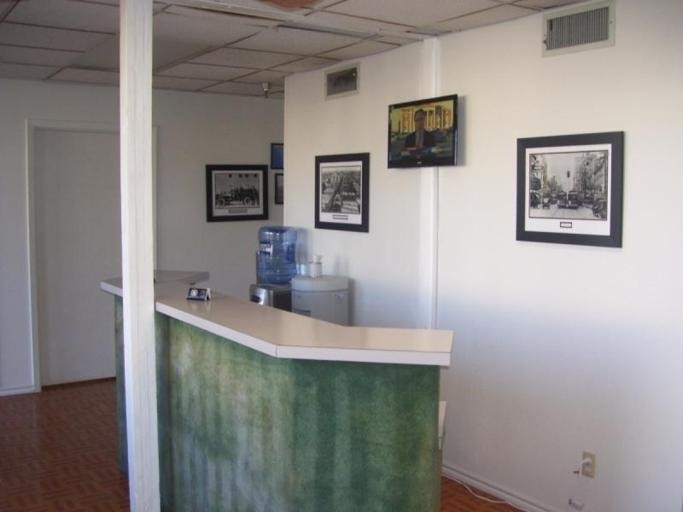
309 262 323 279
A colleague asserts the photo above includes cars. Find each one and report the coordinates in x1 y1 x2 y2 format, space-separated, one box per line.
542 185 606 218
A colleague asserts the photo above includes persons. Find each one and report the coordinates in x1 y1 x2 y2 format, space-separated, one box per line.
405 108 433 149
225 173 259 201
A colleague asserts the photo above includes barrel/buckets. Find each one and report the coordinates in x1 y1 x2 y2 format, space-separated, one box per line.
258 226 298 284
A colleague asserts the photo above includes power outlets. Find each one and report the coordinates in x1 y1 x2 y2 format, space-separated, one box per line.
582 451 595 479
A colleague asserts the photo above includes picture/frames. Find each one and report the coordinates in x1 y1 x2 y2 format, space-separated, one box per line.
314 152 370 233
274 172 283 205
206 163 269 223
270 142 283 170
515 130 624 248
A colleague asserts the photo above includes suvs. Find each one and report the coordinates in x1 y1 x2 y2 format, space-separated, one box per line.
217 185 257 206
